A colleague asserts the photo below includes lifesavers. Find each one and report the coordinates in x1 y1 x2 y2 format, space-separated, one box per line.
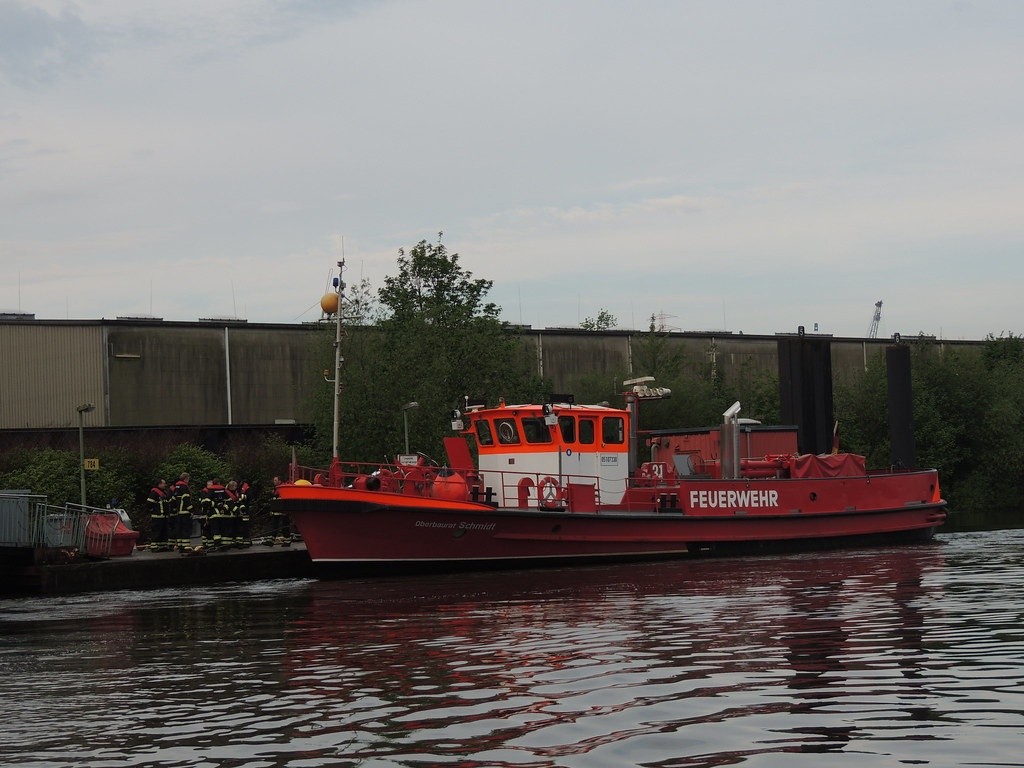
537 477 563 508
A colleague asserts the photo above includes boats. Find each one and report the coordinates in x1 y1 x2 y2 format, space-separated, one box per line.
275 376 948 581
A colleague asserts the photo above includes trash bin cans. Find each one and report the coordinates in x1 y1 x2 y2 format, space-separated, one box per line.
44 514 74 548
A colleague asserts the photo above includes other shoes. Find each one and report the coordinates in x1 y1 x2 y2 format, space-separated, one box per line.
281 543 290 547
151 545 193 553
218 542 249 551
262 541 273 547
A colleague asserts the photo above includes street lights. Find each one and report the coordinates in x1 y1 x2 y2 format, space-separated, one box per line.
76 402 97 513
402 401 421 456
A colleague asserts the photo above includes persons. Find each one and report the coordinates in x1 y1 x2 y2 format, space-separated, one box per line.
262 476 292 547
147 472 252 556
